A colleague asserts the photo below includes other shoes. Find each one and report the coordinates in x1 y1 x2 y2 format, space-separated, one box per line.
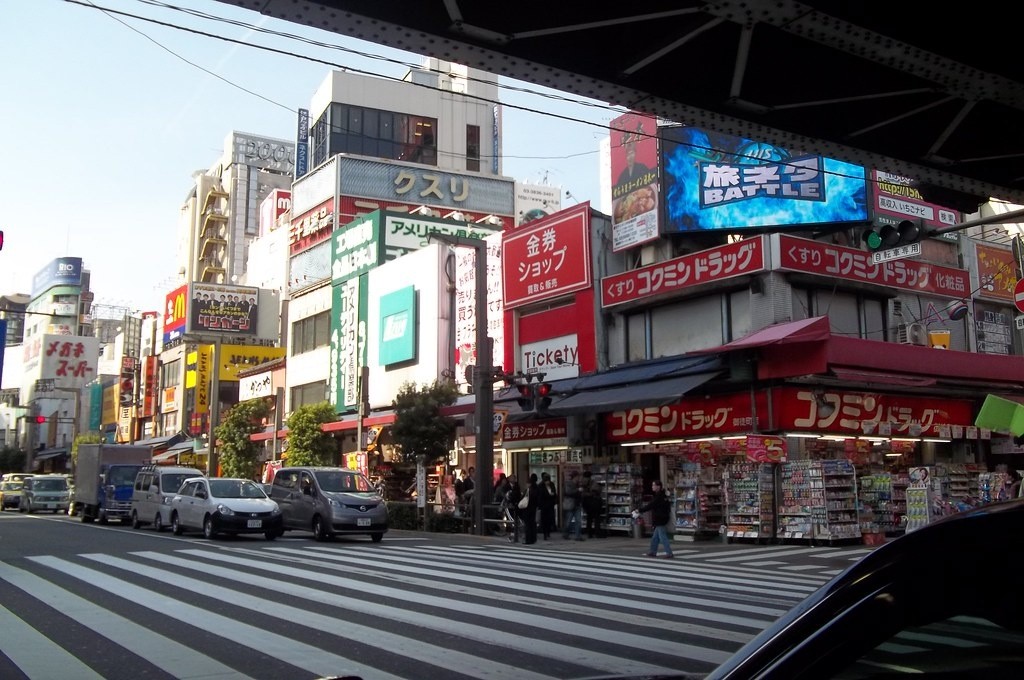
661 553 675 558
645 552 656 556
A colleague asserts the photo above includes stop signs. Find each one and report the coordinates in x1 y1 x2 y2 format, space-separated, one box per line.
1013 277 1024 315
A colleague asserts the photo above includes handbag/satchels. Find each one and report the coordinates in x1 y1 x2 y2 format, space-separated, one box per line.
517 488 530 510
561 496 576 512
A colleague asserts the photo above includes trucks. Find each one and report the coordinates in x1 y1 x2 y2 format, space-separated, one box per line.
74 443 154 524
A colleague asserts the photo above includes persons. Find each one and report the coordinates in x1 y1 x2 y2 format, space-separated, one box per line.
454 467 604 545
617 146 651 184
909 467 928 483
192 292 257 333
632 479 673 559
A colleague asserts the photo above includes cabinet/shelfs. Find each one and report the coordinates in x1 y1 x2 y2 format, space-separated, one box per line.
666 452 1014 546
579 464 642 531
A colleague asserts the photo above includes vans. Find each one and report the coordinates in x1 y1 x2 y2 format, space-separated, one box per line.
269 466 390 542
129 458 204 530
18 477 70 516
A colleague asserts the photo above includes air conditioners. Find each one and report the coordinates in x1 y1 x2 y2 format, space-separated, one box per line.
898 322 928 345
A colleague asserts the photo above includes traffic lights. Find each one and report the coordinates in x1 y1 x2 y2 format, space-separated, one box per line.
859 218 928 250
25 415 45 423
515 383 534 413
533 381 552 412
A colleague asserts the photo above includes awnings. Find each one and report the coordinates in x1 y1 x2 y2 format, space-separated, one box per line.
464 394 564 431
687 315 830 355
549 373 723 418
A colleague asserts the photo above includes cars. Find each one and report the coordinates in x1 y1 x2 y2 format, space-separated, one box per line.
700 500 1024 680
0 480 23 512
167 477 281 541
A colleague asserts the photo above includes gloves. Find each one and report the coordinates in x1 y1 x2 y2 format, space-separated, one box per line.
631 510 642 520
